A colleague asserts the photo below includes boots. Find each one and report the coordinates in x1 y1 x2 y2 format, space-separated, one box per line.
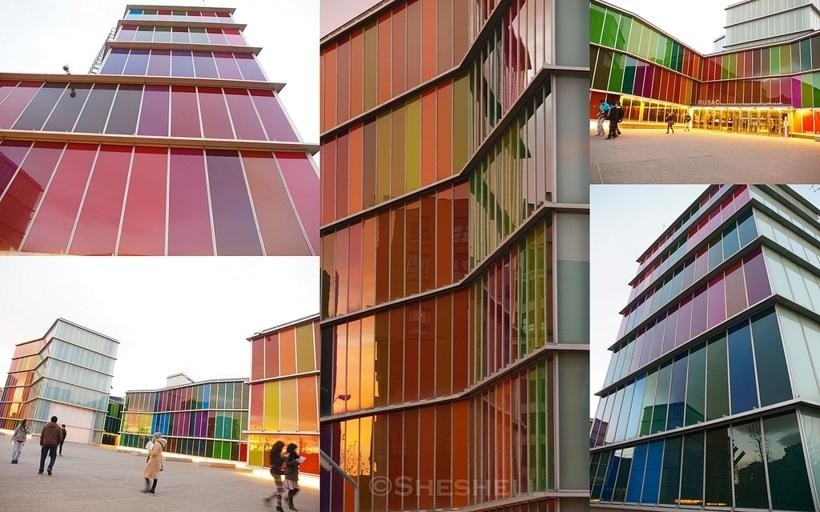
285 488 300 511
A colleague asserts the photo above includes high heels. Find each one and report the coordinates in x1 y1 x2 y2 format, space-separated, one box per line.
276 506 284 512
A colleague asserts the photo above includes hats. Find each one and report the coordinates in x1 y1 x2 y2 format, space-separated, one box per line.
154 431 161 438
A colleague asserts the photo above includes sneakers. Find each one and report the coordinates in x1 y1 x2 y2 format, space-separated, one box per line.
10 458 18 464
595 129 621 140
58 453 64 458
141 487 159 494
39 468 53 476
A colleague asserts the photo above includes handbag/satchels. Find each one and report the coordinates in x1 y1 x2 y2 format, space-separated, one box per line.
160 465 163 471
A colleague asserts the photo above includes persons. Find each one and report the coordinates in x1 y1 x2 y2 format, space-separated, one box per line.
10 419 30 463
684 115 691 132
596 99 624 140
141 431 167 494
666 111 675 135
39 416 63 475
55 424 67 456
264 440 291 512
283 443 305 511
